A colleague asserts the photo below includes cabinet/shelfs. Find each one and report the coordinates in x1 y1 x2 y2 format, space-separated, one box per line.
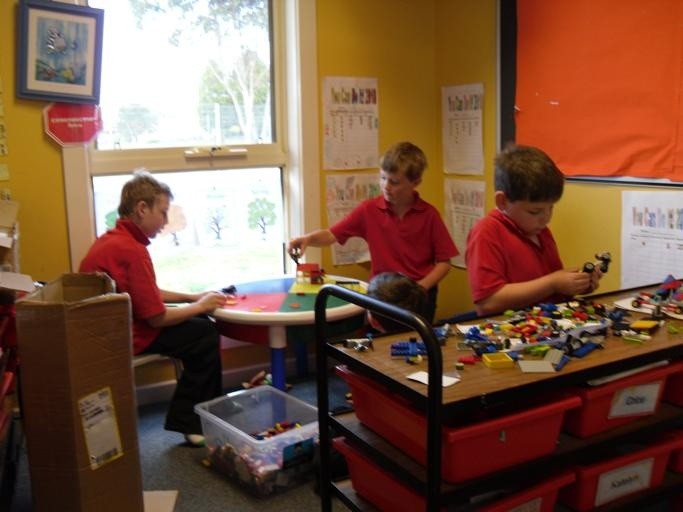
314 280 682 512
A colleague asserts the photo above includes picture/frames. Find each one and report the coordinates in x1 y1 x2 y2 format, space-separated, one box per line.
18 1 104 105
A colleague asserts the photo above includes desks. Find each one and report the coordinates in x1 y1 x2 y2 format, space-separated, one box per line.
211 274 369 428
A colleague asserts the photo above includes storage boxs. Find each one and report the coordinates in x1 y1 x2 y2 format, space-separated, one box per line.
556 439 681 509
194 386 332 499
333 435 577 511
14 272 145 512
562 366 681 436
336 366 584 481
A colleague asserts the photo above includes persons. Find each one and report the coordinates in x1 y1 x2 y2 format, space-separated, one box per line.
77 172 237 448
464 143 606 315
286 138 459 331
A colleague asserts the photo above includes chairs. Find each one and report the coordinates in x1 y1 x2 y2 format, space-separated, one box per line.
131 349 181 390
